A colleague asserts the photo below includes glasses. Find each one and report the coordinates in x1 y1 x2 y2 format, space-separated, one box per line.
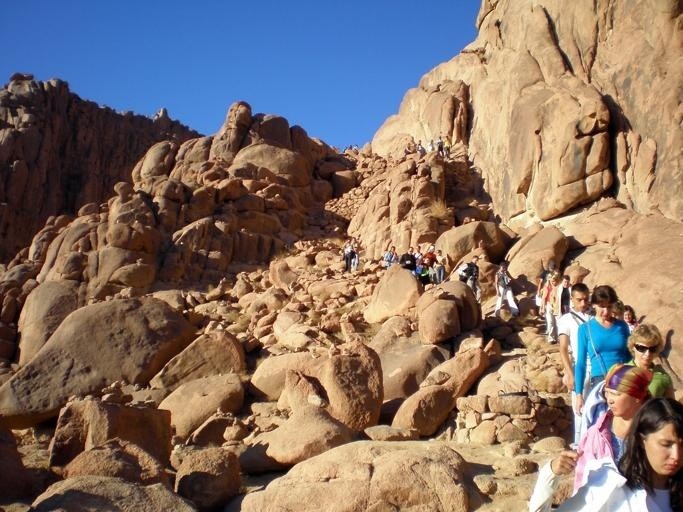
634 343 657 352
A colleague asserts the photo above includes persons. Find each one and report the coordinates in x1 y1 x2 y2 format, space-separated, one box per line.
343 144 358 152
459 255 482 304
341 234 362 273
535 261 683 512
404 136 452 158
382 245 446 284
495 262 520 318
